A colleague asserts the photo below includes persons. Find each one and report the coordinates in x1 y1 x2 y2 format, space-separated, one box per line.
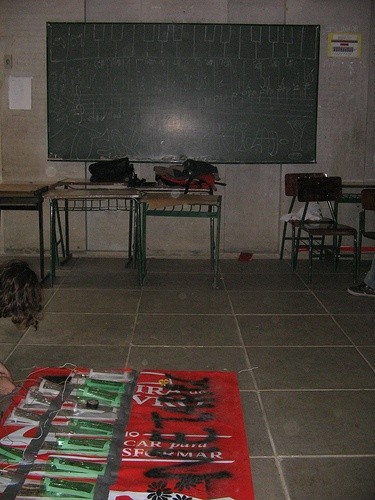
0 257 42 395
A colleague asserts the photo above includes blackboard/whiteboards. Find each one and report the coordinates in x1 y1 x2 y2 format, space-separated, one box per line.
45 21 320 164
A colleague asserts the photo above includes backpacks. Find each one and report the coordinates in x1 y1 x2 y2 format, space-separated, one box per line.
155 174 217 195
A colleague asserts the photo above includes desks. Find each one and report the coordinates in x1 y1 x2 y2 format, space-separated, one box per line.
0 180 222 286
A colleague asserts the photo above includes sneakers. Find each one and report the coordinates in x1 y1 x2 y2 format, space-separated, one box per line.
347 281 375 296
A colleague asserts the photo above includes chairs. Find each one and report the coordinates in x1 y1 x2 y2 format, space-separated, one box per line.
280 173 342 266
296 177 358 282
